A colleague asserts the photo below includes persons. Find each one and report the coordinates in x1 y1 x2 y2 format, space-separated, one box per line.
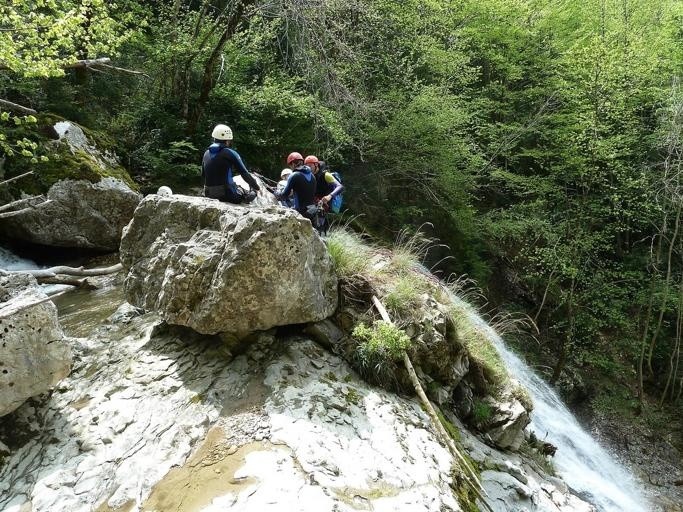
274 151 316 220
277 168 294 208
303 155 343 233
202 124 262 205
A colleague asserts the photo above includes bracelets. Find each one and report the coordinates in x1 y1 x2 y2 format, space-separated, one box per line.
330 192 335 199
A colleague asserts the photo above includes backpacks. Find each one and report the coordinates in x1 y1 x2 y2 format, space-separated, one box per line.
328 171 345 215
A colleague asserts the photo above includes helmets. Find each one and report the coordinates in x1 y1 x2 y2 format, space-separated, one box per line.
281 168 292 179
212 123 233 141
303 153 319 165
286 151 304 165
